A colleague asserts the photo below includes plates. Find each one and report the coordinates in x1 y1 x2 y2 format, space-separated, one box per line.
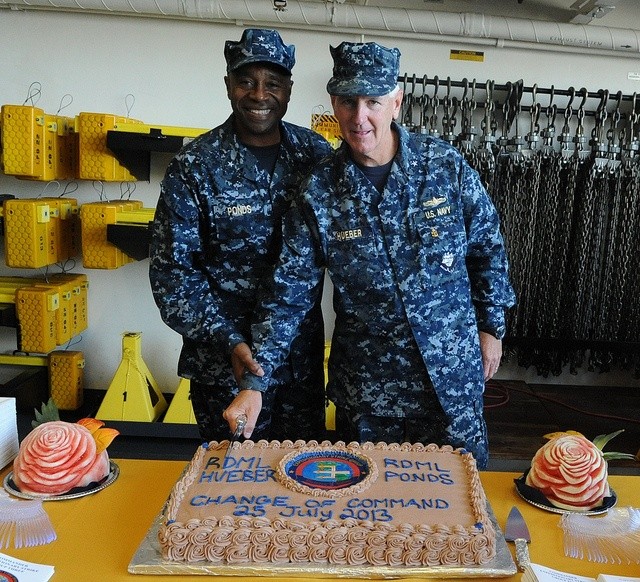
515 473 618 516
3 460 120 501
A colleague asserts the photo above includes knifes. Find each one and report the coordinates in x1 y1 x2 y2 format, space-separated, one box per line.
220 415 247 469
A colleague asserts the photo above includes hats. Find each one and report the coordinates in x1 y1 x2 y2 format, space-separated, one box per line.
223 26 296 76
326 40 401 99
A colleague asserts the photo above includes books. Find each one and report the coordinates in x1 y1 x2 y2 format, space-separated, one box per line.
1 552 55 582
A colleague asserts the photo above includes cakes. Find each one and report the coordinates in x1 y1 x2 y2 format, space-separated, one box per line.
525 434 615 513
157 440 498 565
11 420 112 497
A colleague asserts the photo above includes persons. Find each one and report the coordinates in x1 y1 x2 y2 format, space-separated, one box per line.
148 28 336 443
222 41 517 470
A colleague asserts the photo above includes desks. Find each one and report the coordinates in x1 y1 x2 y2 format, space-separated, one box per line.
0 412 637 580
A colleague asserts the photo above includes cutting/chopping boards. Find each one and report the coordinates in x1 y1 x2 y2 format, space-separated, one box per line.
128 463 518 578
